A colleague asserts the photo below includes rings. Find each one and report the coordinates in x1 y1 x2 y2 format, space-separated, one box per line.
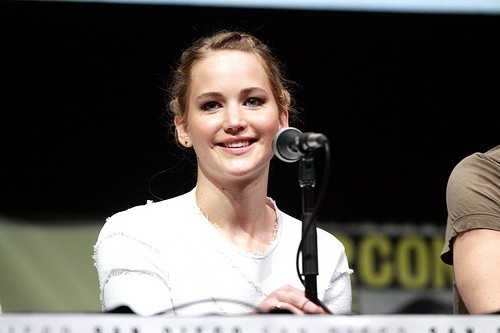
276 301 282 308
300 299 308 310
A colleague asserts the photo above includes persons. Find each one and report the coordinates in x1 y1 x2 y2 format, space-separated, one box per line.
440 145 500 316
93 31 354 314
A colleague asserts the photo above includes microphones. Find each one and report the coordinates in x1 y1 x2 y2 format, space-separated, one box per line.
272 127 328 163
